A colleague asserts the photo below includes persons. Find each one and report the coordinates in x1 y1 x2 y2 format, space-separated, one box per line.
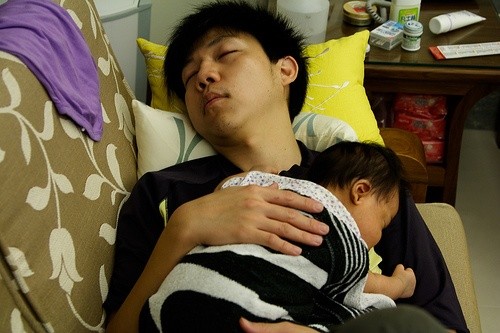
147 140 417 332
102 0 471 333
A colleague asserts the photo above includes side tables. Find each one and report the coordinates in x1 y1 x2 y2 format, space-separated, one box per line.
324 0 500 208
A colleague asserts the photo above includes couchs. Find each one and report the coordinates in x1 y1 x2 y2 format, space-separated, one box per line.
0 0 483 333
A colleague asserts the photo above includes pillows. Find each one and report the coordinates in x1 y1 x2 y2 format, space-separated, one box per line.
131 99 357 181
135 29 386 148
